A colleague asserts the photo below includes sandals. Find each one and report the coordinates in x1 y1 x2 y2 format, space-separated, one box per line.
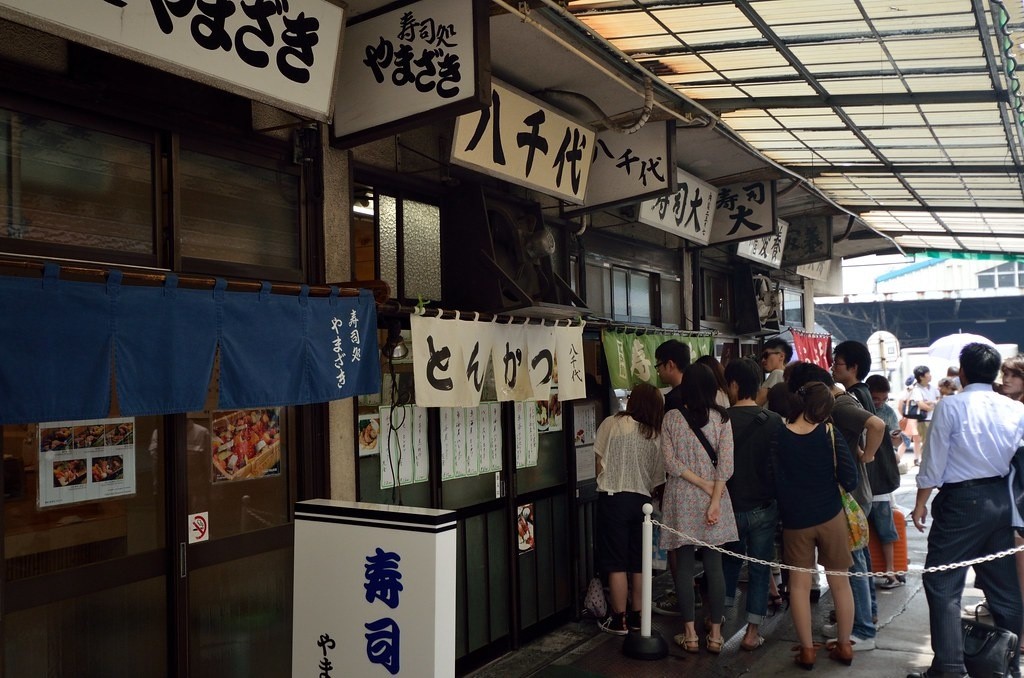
706 633 724 652
673 633 699 653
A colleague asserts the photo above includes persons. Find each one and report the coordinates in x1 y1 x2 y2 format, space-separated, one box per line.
898 366 961 466
654 339 906 616
725 358 783 649
906 342 1024 678
660 364 741 652
963 354 1024 656
593 384 667 635
768 382 859 670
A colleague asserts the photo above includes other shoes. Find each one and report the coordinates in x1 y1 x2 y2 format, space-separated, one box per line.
964 598 991 615
737 562 901 670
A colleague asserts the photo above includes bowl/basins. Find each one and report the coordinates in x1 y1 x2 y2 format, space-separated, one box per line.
92 455 123 482
53 459 87 487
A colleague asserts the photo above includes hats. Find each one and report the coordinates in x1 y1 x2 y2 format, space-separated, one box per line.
904 375 917 388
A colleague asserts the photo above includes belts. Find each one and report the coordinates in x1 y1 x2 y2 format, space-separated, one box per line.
938 475 1005 490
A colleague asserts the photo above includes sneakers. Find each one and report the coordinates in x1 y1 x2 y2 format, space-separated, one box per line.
652 588 702 616
627 609 643 631
597 610 628 635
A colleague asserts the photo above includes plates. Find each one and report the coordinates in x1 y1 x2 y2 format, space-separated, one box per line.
537 422 549 431
359 431 380 451
518 522 533 551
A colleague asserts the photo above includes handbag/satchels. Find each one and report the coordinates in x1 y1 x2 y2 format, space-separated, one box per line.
902 387 928 419
584 578 607 617
838 483 870 552
865 425 900 495
960 604 1019 678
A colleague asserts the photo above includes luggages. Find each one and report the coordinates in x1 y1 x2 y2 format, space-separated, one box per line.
867 507 907 583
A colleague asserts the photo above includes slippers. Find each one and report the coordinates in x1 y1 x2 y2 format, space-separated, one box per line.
740 633 765 652
703 615 726 629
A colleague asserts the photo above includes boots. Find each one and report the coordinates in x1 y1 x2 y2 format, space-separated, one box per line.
914 460 920 466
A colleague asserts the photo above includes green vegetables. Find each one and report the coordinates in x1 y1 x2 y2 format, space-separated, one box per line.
536 403 541 413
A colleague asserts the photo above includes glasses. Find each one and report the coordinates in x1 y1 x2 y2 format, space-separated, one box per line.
654 360 676 372
760 352 779 361
873 398 889 403
627 395 631 399
832 362 847 367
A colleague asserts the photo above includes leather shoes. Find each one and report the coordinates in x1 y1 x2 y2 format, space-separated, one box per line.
906 672 971 678
